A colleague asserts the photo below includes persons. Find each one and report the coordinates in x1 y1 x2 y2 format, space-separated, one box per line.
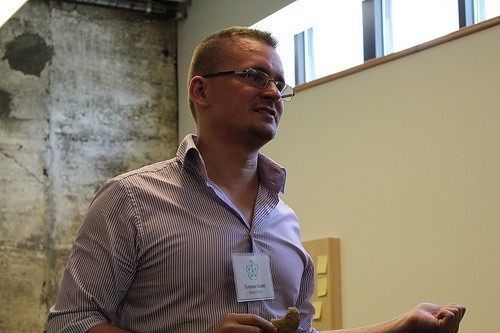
42 27 467 333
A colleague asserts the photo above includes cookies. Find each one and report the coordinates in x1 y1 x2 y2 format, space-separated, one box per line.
271 307 300 333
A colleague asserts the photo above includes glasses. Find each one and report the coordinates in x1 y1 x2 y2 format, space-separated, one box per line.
201 67 295 102
